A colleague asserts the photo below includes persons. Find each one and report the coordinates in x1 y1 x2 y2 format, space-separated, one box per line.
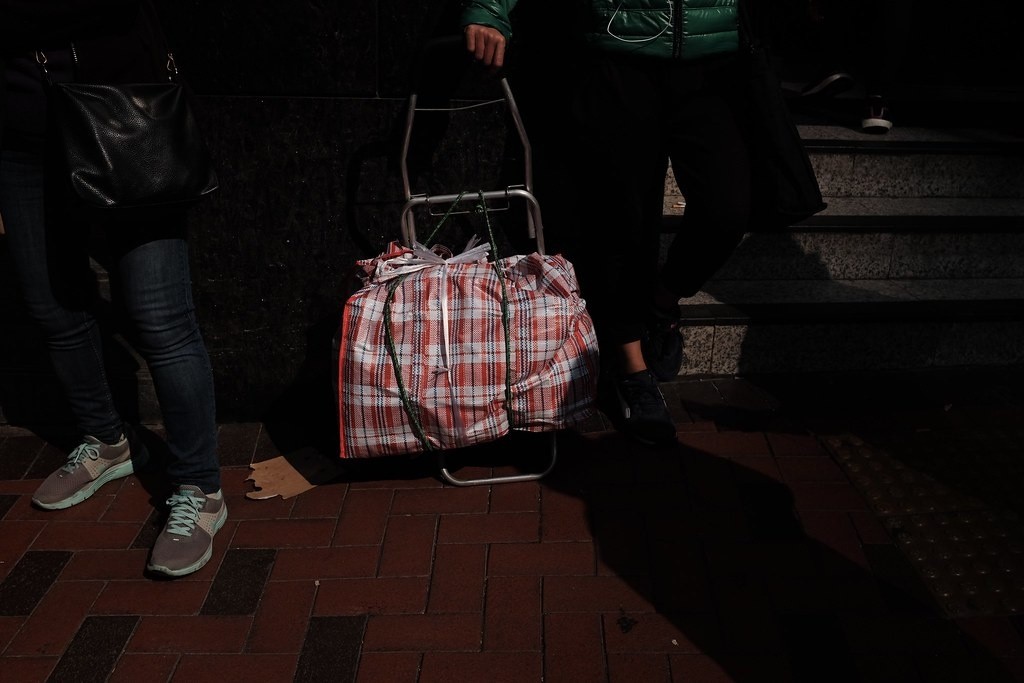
0 0 228 576
455 0 914 445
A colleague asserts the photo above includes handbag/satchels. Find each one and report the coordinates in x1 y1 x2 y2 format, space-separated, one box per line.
735 0 828 232
339 244 600 460
43 64 220 210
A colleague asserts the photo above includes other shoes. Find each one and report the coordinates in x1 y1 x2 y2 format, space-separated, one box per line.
857 71 894 130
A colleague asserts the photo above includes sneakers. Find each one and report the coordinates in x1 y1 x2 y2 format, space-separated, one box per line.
616 371 678 444
32 426 149 510
147 484 228 576
641 293 685 379
